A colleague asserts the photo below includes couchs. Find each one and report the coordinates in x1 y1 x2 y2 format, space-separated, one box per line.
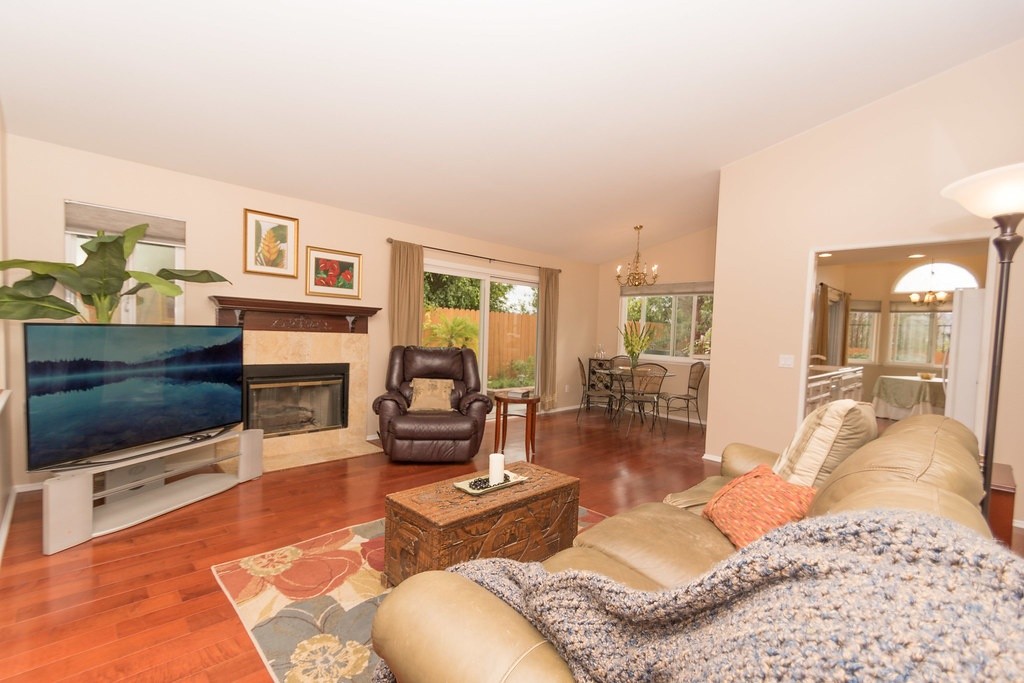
373 345 493 461
372 414 994 683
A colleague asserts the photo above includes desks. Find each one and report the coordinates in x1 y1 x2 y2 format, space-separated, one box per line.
872 375 948 421
595 370 675 425
494 392 540 457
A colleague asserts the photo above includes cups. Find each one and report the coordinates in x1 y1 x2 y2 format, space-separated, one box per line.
489 453 504 485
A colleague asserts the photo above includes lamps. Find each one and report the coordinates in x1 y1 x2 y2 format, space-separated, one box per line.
615 225 658 286
909 257 947 306
941 161 1024 522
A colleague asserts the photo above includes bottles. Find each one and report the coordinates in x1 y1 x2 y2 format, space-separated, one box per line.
595 344 603 358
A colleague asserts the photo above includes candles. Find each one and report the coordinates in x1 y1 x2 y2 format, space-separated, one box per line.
489 451 504 485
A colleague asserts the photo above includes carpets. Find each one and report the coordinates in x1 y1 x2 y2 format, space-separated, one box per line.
211 506 609 682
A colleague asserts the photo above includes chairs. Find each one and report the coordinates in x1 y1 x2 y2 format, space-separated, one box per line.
576 355 707 439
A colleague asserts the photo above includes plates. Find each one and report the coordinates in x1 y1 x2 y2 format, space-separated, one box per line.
454 470 529 495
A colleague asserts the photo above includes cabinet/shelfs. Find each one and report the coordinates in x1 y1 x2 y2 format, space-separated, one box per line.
805 373 863 415
43 429 264 555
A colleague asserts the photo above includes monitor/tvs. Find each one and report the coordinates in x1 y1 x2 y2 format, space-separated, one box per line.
22 322 245 473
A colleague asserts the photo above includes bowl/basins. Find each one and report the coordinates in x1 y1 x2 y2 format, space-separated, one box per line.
917 373 937 380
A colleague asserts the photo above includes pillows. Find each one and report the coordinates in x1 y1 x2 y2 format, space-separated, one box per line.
772 399 878 488
407 378 458 412
702 464 820 552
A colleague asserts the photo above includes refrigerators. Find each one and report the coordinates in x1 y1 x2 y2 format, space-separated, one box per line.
941 288 986 434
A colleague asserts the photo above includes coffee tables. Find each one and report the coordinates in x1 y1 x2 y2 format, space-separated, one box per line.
382 461 581 586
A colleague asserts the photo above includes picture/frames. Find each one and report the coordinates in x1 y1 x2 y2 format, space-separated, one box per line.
243 208 299 279
305 246 362 300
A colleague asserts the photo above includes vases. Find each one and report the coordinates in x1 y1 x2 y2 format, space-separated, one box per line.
629 356 638 368
